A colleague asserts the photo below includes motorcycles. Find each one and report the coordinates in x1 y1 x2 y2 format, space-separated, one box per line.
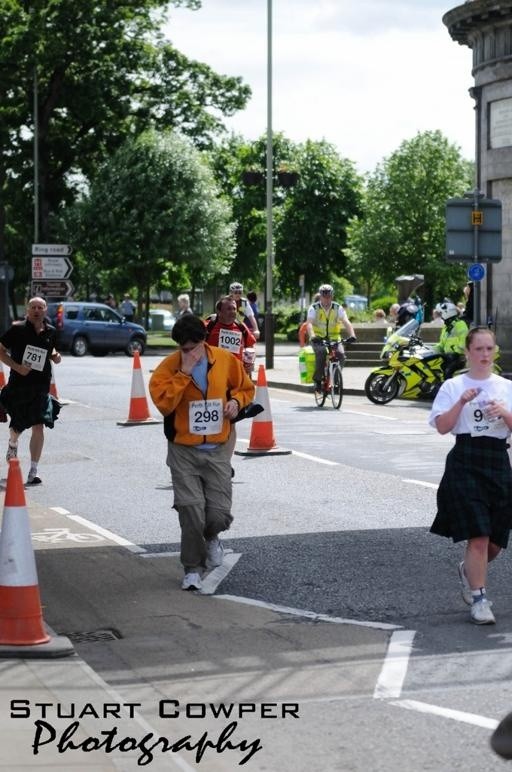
364 319 502 403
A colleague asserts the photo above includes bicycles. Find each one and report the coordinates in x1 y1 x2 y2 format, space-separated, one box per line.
239 328 256 383
313 337 350 411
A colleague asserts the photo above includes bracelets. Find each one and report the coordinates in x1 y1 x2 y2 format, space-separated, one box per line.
231 398 239 409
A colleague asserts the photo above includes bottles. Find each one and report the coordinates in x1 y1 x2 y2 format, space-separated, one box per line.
476 387 501 422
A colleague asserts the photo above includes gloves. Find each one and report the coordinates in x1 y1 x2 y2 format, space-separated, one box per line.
312 335 323 344
344 336 355 346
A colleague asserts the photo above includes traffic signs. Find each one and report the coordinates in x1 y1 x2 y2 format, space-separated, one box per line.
32 280 75 299
33 245 72 256
32 257 74 279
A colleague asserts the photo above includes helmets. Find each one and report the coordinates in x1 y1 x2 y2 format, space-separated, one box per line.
438 303 458 320
319 284 334 296
230 282 244 292
397 303 418 315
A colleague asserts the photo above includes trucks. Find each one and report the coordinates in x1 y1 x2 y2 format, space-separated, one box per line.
340 294 367 311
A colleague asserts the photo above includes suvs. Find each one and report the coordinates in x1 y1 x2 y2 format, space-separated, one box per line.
46 301 148 357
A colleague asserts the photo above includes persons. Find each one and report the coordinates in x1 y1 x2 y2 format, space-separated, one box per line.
174 282 260 479
433 302 469 381
308 285 356 394
121 293 136 322
0 296 62 484
148 314 255 591
427 326 512 625
384 303 421 358
104 295 116 309
371 286 472 325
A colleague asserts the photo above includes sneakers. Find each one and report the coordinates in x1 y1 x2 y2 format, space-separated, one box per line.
470 599 496 625
27 474 42 483
207 539 225 567
6 446 17 461
457 561 473 605
313 382 320 391
182 572 202 590
